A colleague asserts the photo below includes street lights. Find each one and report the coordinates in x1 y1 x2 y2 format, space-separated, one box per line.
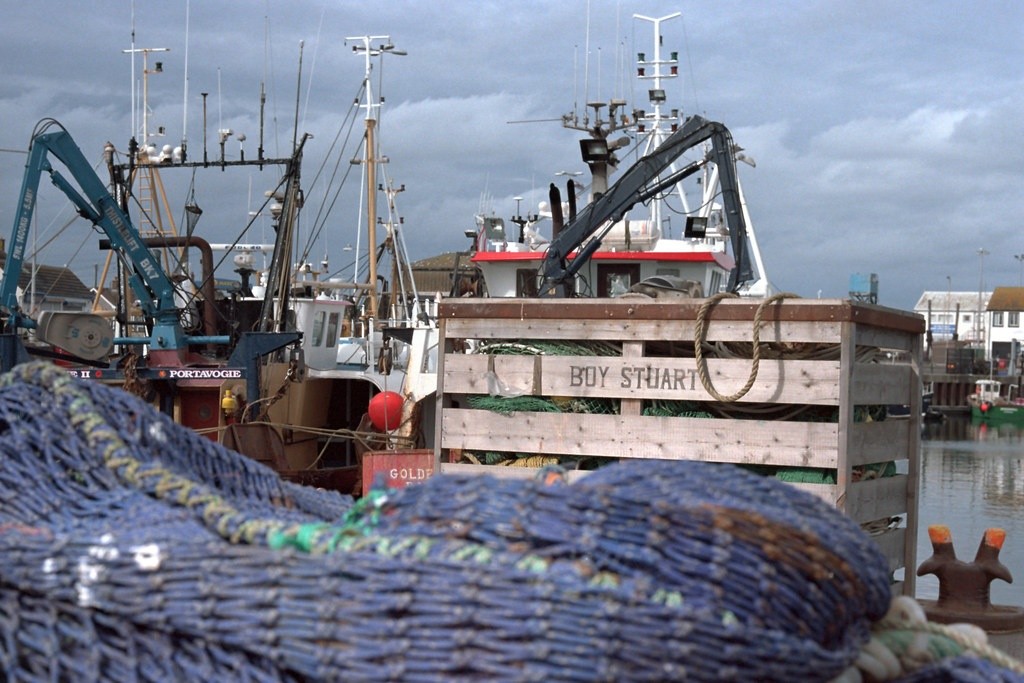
977 247 991 346
